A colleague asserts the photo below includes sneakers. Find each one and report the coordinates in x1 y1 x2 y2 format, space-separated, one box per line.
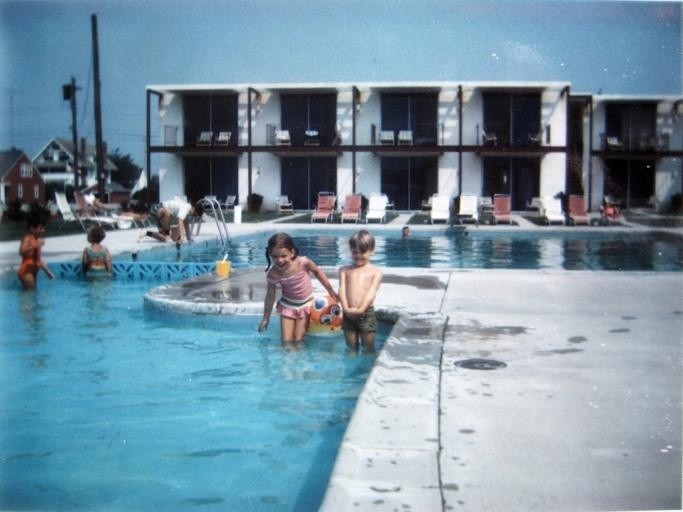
136 229 146 243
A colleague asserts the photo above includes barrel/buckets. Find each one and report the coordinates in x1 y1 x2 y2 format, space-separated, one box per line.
216 261 230 276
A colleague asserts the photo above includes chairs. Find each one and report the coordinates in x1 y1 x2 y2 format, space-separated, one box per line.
308 191 389 225
194 131 231 147
377 130 413 145
539 194 625 228
426 194 513 225
51 189 151 233
273 195 294 215
202 196 236 214
274 130 320 147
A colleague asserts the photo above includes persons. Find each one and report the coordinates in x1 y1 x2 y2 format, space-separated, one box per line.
138 198 204 247
82 226 114 281
337 229 384 355
92 196 150 224
257 231 340 344
401 226 412 239
19 211 55 289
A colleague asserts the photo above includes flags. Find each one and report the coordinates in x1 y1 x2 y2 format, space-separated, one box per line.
129 169 148 199
